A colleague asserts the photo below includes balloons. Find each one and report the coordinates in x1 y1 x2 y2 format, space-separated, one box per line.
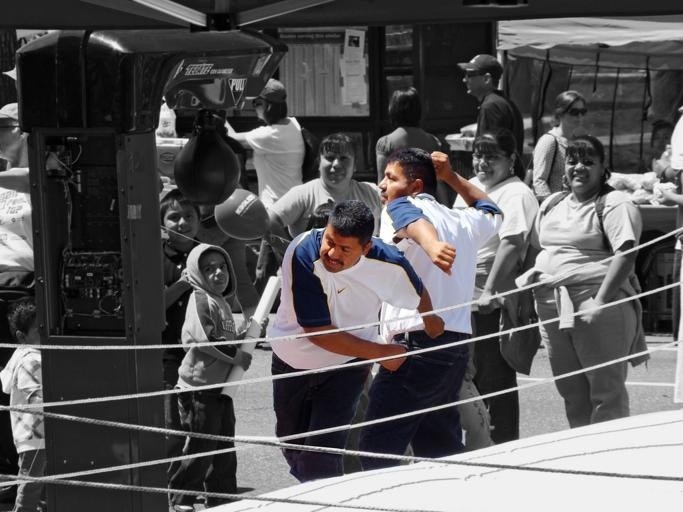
215 188 271 240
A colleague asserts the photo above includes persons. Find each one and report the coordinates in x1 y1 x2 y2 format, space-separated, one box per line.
226 78 305 311
263 197 444 484
532 91 587 201
159 191 213 484
528 135 644 431
0 305 51 510
457 54 526 182
356 144 505 469
263 133 383 257
375 87 447 203
165 241 254 509
660 109 682 345
421 146 495 452
447 126 540 446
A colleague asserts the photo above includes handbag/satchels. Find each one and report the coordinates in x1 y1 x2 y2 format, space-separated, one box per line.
301 127 318 168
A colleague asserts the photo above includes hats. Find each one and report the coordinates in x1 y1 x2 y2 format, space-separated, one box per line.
0 103 20 121
259 77 286 103
456 53 503 78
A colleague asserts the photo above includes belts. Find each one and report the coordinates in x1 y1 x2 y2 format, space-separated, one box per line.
393 330 470 341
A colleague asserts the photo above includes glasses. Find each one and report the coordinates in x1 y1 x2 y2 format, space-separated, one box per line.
566 107 586 116
251 99 263 108
